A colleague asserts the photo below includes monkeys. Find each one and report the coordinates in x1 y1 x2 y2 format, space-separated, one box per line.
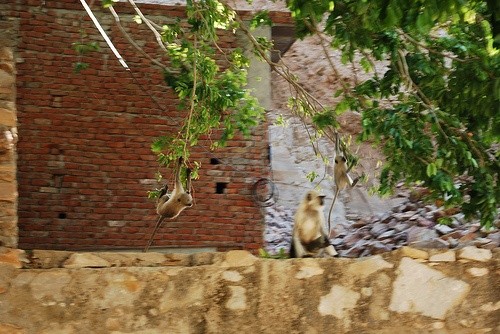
156 155 193 218
292 191 339 259
336 129 361 191
